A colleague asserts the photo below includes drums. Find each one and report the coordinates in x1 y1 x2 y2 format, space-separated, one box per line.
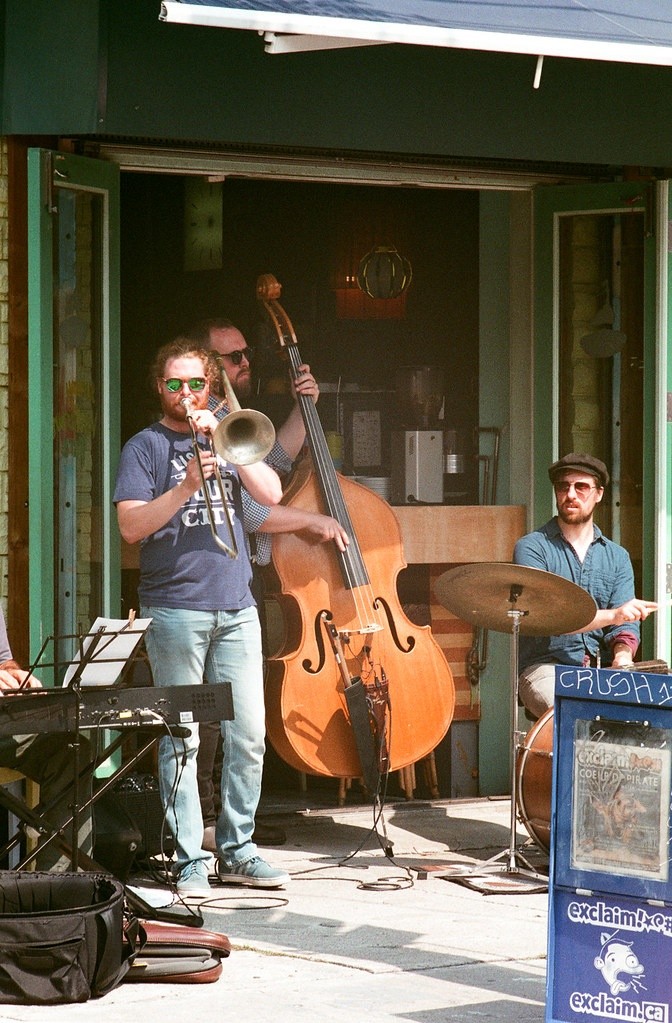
514 703 560 856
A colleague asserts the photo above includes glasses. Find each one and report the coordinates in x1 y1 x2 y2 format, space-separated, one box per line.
554 480 597 495
162 376 208 393
221 346 256 365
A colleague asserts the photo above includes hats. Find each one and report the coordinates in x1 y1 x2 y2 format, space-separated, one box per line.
548 452 610 487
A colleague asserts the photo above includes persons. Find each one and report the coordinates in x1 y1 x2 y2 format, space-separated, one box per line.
509 452 661 722
107 336 289 900
195 321 351 851
1 599 98 873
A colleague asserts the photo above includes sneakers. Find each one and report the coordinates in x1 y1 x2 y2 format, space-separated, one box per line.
176 860 212 898
217 856 291 887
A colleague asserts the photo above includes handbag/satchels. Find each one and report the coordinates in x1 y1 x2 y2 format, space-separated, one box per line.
0 867 146 1007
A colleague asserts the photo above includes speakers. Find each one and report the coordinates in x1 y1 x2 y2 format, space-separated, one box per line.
536 663 671 1023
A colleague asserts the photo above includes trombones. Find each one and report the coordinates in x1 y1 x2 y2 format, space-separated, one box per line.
178 349 276 562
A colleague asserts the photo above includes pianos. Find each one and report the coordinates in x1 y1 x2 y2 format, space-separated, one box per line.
0 678 234 735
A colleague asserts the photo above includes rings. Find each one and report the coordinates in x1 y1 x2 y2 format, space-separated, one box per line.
313 378 317 384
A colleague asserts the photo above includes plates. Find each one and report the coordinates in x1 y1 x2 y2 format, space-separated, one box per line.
356 476 391 503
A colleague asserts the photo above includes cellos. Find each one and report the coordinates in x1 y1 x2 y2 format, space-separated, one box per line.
247 269 456 803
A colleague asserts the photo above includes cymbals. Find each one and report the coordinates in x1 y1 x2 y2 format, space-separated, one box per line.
435 561 598 637
